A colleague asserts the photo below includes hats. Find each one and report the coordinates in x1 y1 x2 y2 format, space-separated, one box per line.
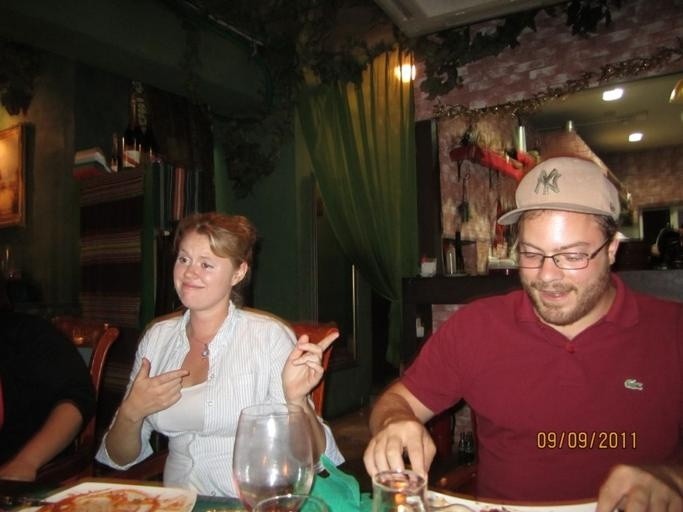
497 156 621 226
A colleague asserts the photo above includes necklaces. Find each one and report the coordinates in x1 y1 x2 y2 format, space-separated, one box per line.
191 330 215 355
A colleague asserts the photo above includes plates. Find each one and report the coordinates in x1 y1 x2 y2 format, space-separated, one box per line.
14 482 198 512
418 490 616 512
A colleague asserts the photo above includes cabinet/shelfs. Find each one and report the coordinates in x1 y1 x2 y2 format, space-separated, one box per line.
76 162 200 397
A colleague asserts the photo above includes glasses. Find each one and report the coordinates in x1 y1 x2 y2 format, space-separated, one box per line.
519 234 610 269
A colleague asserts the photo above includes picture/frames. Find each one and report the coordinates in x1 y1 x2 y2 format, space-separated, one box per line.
0 120 30 228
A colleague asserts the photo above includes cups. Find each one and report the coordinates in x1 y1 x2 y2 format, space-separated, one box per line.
420 256 437 277
232 403 313 511
248 494 331 512
122 149 140 168
370 465 431 512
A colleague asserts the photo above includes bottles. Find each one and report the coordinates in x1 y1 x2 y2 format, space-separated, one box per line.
445 243 456 277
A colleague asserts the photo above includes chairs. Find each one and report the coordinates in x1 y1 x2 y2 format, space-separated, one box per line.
1 313 120 495
104 319 342 480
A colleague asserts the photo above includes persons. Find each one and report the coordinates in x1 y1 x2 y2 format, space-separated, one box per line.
362 157 683 511
94 210 346 498
0 307 100 486
